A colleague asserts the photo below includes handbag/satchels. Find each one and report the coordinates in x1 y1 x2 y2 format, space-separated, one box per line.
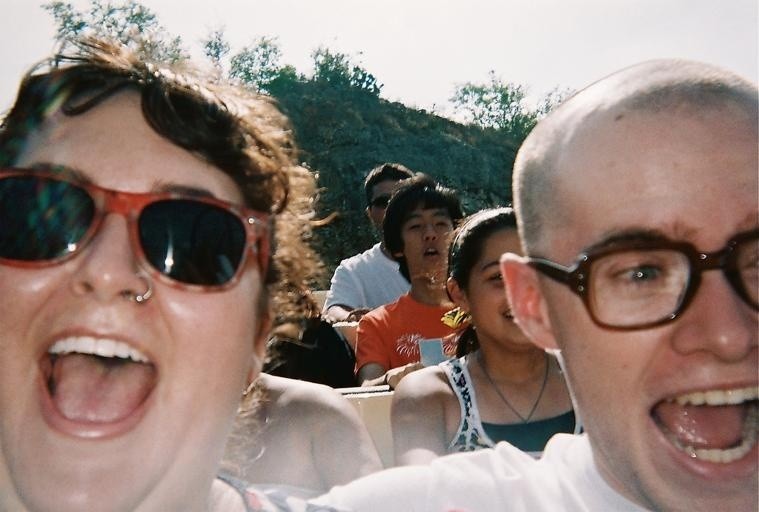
267 313 357 388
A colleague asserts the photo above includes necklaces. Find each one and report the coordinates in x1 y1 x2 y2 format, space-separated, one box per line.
477 349 550 424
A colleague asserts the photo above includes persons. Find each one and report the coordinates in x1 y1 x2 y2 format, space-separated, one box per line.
355 171 474 387
261 282 357 388
217 372 385 492
0 38 333 512
389 208 585 466
305 59 758 511
322 162 415 320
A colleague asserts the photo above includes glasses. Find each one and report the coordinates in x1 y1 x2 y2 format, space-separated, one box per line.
527 228 758 330
1 172 270 292
369 196 388 209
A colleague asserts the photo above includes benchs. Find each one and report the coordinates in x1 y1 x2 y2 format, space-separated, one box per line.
311 290 395 470
